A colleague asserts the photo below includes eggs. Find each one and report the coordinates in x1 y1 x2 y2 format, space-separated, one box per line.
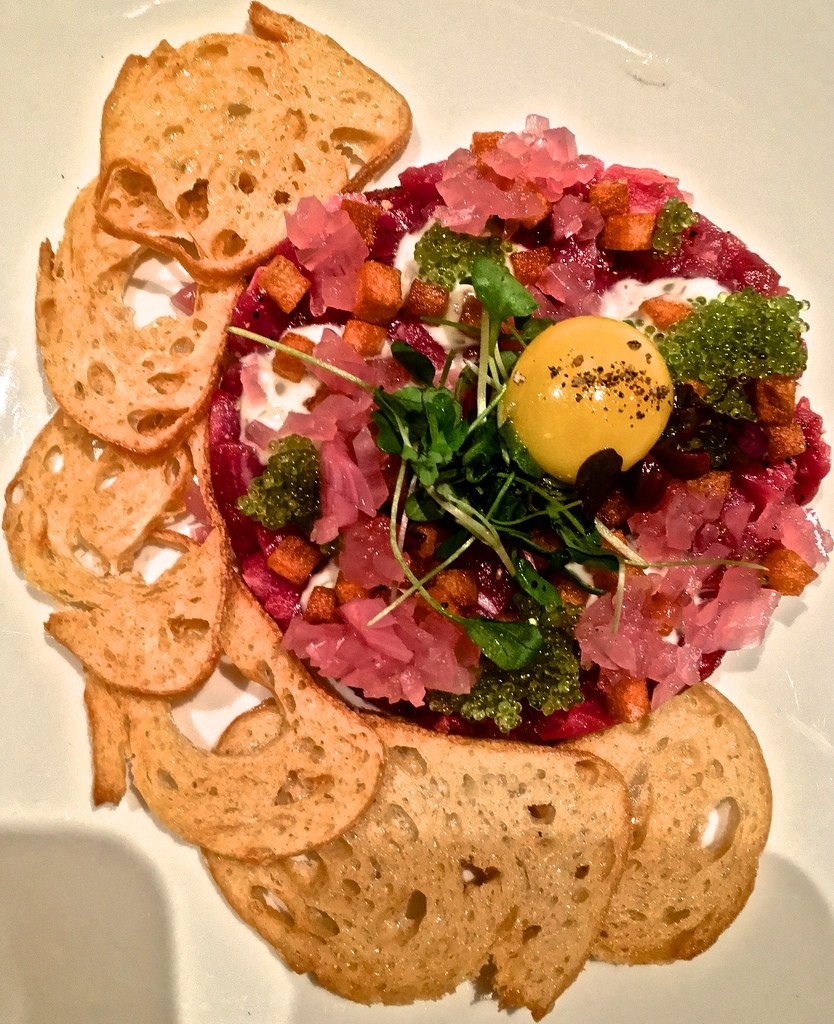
498 313 676 482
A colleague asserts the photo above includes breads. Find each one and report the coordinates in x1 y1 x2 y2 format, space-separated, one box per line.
202 698 633 1022
1 401 233 697
545 680 772 967
88 2 413 286
35 174 251 453
82 567 387 861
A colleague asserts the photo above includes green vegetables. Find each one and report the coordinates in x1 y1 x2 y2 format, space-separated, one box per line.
228 253 776 676
